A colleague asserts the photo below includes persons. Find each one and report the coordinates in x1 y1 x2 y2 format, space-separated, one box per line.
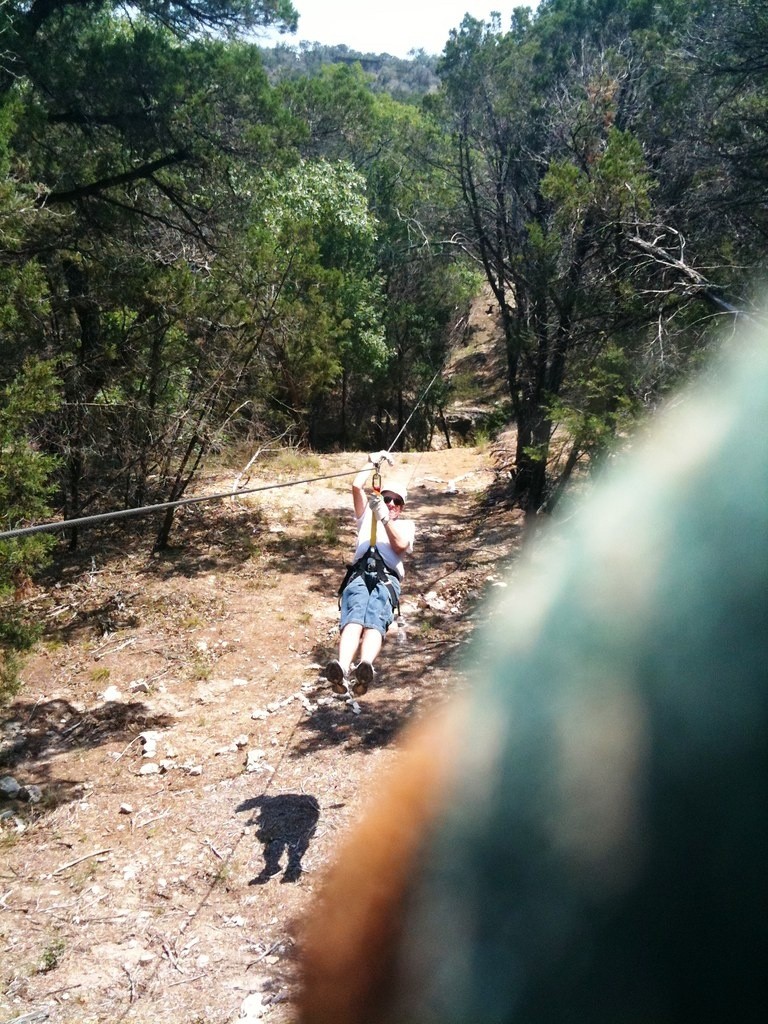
325 450 415 695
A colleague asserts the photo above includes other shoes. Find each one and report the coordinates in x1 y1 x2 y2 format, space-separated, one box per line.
326 660 348 694
351 661 374 695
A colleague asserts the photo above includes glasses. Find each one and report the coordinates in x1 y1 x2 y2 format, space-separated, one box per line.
383 496 402 505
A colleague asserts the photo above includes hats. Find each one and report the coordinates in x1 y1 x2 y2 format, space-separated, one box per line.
380 481 407 504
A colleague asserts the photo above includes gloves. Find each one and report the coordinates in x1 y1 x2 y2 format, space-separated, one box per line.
368 493 389 520
368 449 393 467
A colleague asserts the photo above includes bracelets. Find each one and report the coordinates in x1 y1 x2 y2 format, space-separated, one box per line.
382 515 391 526
